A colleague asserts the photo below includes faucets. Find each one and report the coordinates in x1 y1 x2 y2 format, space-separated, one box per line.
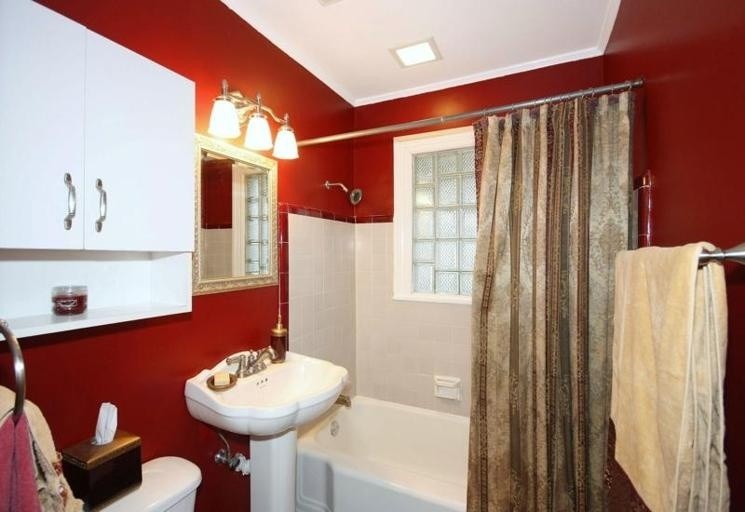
248 346 279 374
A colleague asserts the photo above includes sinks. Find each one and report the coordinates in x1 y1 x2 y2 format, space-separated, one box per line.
184 346 348 436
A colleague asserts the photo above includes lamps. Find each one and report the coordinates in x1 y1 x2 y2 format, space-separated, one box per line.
206 76 300 162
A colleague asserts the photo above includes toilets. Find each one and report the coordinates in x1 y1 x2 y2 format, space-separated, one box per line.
88 456 204 512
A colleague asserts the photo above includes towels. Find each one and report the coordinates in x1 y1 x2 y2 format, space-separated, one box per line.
609 237 736 512
0 386 87 512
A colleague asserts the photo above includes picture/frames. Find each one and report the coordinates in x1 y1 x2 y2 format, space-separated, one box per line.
192 131 279 299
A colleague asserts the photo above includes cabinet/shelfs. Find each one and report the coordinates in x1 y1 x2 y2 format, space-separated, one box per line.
1 0 199 350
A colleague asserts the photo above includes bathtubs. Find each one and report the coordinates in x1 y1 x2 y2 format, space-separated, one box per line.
295 395 470 512
